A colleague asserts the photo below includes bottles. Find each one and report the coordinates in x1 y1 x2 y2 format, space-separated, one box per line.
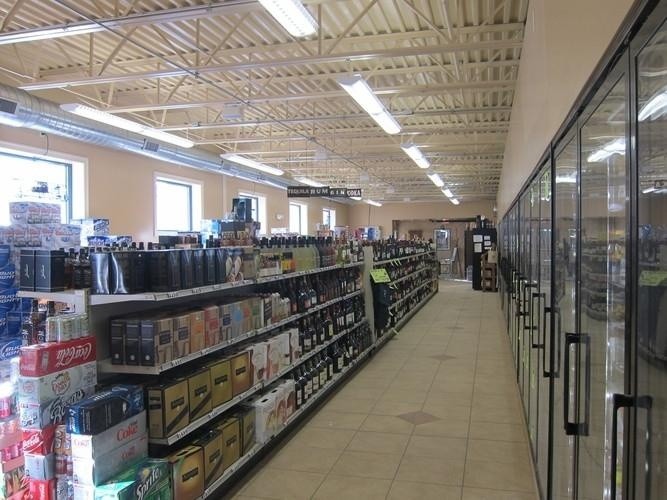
207 233 434 412
64 247 91 291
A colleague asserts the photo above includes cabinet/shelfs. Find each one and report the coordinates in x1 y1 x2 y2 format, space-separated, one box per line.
565 232 666 372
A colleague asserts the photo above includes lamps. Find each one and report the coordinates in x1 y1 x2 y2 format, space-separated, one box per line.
220 153 283 178
399 143 429 169
427 172 459 207
338 74 401 135
259 1 318 39
59 102 194 150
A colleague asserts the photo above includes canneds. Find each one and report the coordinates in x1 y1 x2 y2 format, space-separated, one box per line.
54 425 75 500
0 358 28 496
45 313 89 342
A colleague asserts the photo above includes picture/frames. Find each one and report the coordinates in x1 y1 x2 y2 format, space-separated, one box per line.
433 228 451 251
